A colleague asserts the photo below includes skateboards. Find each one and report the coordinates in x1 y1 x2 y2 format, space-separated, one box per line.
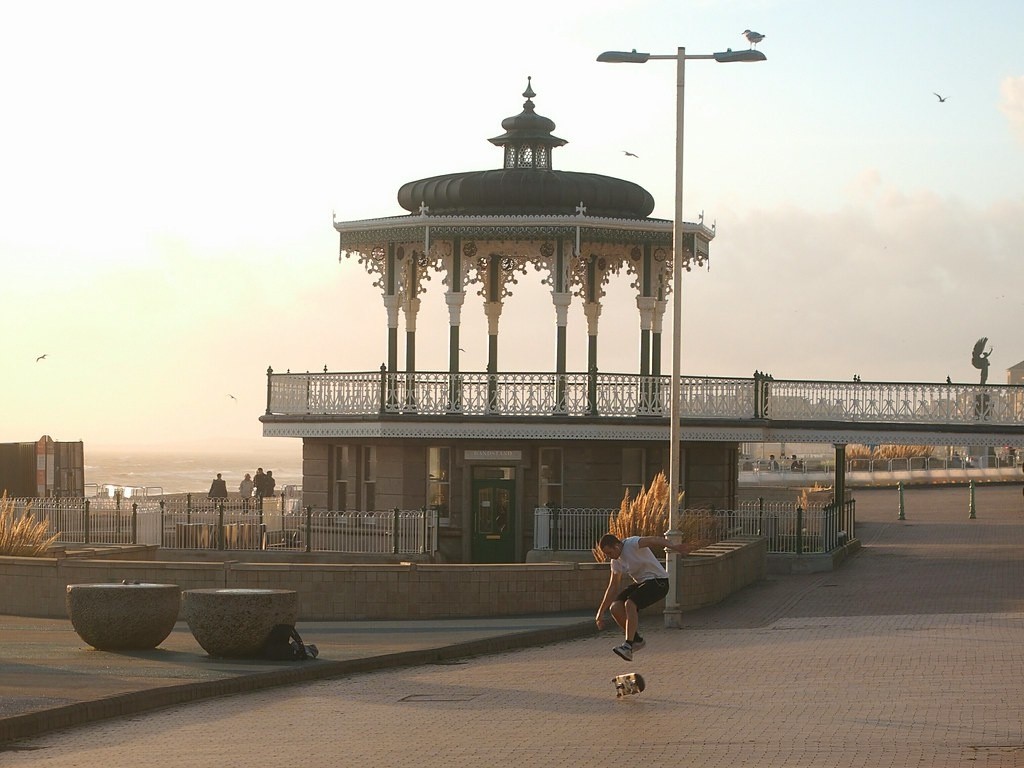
610 672 645 698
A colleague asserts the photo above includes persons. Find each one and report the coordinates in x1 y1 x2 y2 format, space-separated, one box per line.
238 468 275 513
209 474 227 502
953 450 959 462
596 535 693 661
742 455 805 471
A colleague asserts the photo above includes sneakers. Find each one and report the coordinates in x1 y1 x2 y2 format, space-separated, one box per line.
632 638 646 653
613 642 633 661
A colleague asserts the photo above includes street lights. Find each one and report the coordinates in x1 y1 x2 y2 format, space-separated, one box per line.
594 47 770 627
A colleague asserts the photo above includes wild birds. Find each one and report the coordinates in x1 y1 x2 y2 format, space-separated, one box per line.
935 93 950 103
227 393 236 400
36 354 47 361
742 29 765 50
620 149 640 159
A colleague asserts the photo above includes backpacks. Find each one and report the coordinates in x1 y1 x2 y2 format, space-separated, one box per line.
264 622 308 662
